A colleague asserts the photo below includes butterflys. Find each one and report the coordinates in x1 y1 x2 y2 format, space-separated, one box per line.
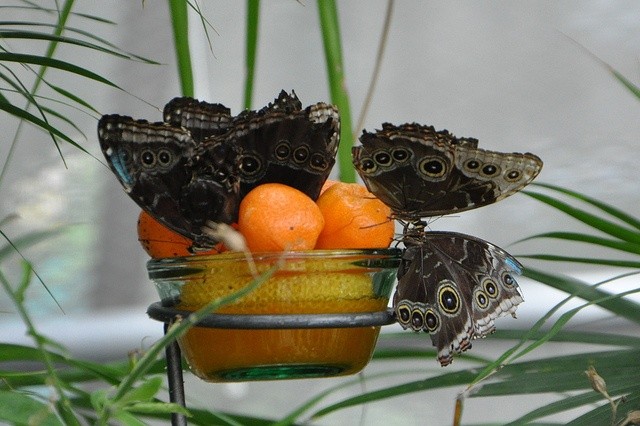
386 211 527 367
96 100 243 257
202 100 342 202
158 89 303 144
349 122 543 230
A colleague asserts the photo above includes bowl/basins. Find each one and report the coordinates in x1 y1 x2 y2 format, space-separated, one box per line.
145 247 407 382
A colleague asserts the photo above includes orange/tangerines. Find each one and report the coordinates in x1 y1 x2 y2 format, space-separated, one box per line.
136 207 196 259
237 182 326 253
196 221 241 257
317 177 337 197
316 181 396 250
